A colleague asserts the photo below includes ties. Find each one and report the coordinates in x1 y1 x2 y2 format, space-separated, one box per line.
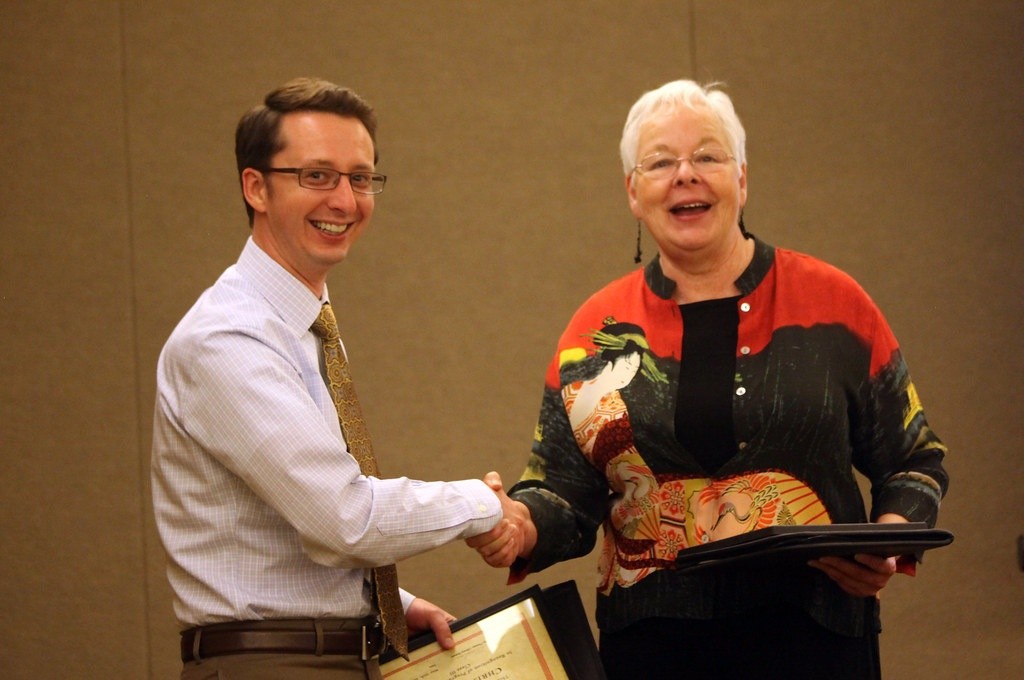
309 303 410 663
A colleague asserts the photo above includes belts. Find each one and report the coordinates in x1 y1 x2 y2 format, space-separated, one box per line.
180 627 385 662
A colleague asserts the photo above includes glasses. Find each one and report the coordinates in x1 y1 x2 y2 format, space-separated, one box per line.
631 148 736 180
258 167 387 195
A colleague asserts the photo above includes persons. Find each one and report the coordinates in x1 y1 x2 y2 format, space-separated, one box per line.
463 78 951 680
151 76 528 680
